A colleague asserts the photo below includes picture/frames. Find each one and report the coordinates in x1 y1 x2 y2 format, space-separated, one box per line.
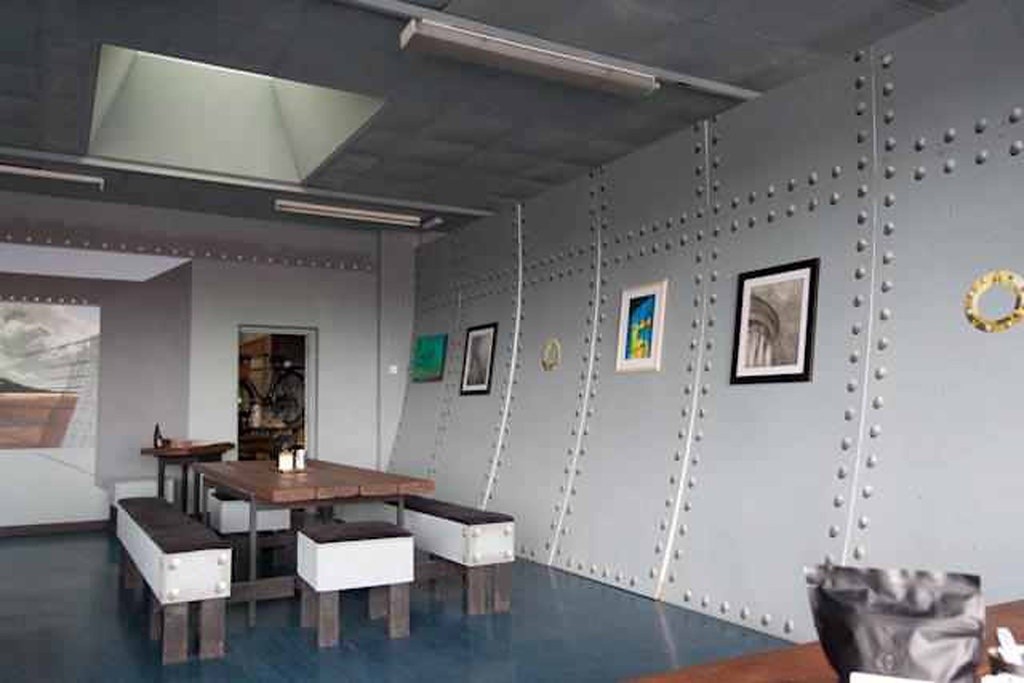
411 332 448 382
615 280 668 372
460 322 498 395
729 256 821 386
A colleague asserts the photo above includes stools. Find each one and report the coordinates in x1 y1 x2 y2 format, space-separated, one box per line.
295 521 415 648
212 493 290 568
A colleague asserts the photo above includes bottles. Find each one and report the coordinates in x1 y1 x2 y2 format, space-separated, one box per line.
153 424 163 448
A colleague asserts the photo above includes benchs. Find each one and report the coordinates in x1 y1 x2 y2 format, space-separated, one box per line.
115 498 233 664
381 496 515 616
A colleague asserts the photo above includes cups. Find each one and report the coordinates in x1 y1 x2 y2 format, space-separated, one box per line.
989 645 1024 675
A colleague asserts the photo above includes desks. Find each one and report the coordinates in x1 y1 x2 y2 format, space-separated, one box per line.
140 442 235 513
617 599 1024 683
192 459 435 629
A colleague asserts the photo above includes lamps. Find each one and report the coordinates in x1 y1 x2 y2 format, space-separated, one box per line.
274 201 422 228
399 18 661 101
